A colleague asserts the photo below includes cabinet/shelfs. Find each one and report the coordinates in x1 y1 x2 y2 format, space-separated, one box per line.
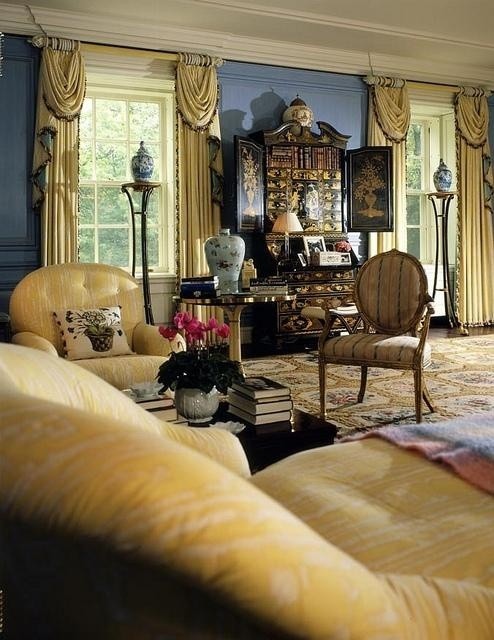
233 94 394 347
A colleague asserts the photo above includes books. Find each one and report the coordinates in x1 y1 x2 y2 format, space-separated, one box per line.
181 277 221 298
268 145 341 169
249 278 289 296
225 377 293 424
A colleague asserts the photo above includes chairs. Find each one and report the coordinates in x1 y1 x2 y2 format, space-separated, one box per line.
9 262 186 394
318 249 437 424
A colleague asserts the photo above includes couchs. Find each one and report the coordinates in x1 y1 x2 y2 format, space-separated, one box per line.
0 343 494 638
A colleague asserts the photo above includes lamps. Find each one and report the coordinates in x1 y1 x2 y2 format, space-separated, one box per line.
272 214 304 260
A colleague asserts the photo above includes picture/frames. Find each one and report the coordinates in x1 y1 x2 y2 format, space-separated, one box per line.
303 236 326 266
297 253 307 266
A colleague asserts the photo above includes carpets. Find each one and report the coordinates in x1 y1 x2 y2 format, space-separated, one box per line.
241 334 494 442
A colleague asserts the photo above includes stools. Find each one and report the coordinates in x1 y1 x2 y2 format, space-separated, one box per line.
300 303 366 339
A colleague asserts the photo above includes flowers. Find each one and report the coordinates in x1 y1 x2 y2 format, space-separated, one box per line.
154 311 244 397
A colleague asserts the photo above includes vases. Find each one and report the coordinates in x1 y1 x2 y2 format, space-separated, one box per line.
175 389 219 424
204 229 246 291
433 158 453 191
131 140 154 181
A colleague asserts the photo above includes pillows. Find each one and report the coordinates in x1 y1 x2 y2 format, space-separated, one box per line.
51 304 133 360
